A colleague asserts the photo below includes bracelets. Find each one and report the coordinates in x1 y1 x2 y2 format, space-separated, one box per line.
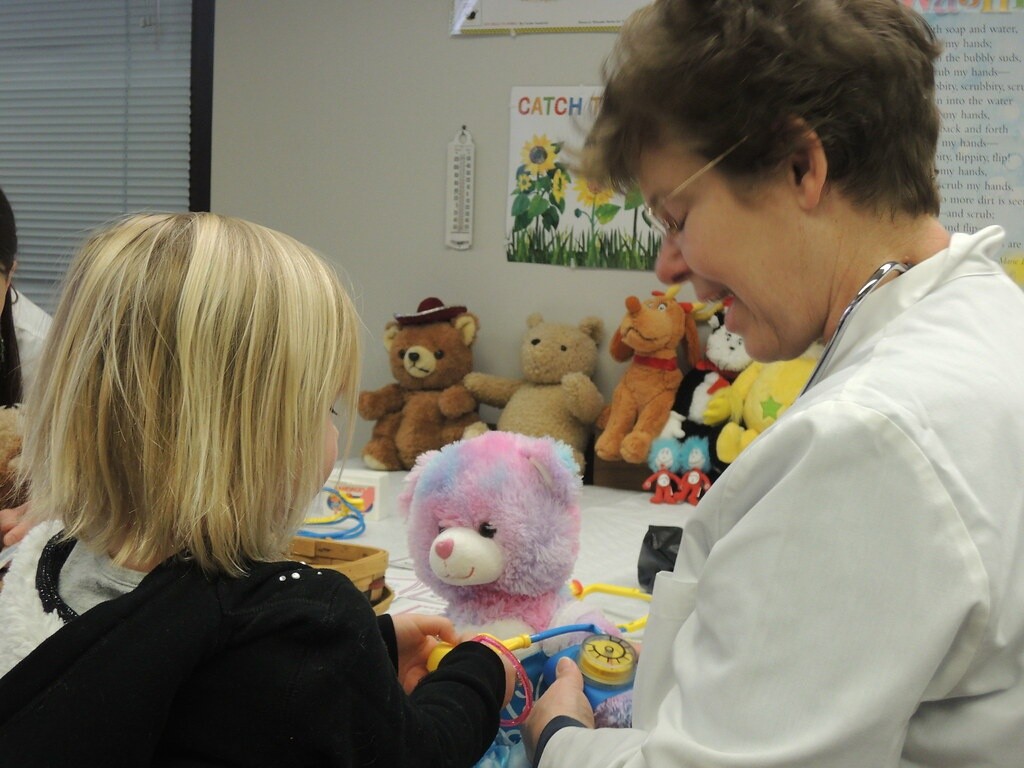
473 636 533 728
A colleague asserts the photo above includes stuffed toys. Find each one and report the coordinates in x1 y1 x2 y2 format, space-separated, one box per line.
464 312 602 480
359 296 481 471
396 430 641 728
594 281 707 461
642 436 712 506
673 303 820 463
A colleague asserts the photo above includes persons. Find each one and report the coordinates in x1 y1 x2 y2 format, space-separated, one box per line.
0 213 533 768
526 0 1024 767
0 191 72 545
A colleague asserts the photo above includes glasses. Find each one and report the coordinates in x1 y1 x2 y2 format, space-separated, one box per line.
639 132 748 241
